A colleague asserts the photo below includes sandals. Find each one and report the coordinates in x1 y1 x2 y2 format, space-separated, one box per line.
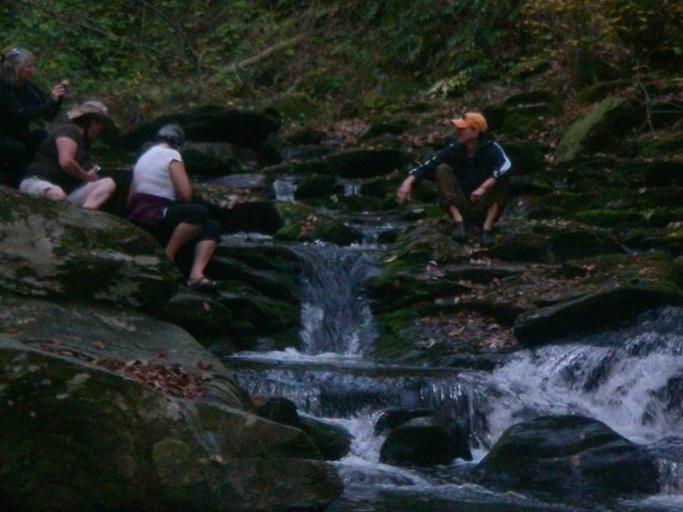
185 275 221 291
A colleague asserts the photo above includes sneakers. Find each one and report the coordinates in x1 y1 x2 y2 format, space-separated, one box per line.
451 223 465 238
481 230 493 247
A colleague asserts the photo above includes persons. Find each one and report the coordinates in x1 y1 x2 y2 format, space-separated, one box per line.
0 46 70 193
395 113 515 245
124 122 222 289
17 99 118 213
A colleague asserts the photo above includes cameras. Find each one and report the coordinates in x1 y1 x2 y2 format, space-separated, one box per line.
95 165 104 177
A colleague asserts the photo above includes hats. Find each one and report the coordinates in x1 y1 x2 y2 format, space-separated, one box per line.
66 101 120 133
452 113 486 132
